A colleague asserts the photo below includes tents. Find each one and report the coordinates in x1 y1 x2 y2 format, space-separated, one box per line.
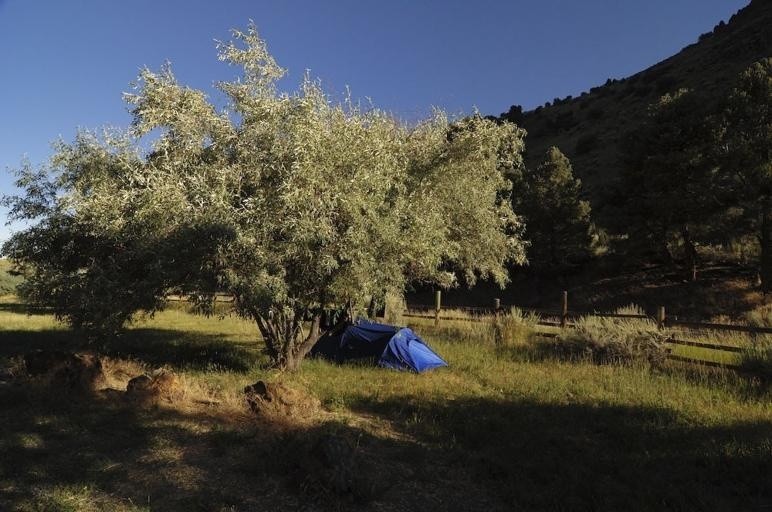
308 315 450 377
